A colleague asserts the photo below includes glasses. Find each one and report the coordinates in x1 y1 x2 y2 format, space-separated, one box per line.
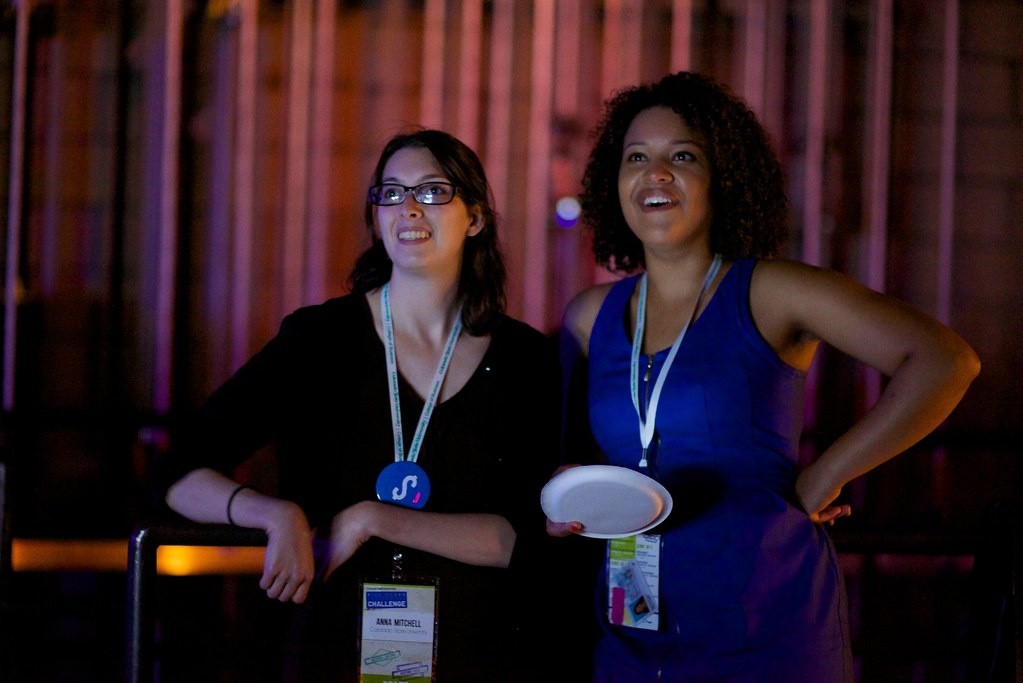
369 182 459 206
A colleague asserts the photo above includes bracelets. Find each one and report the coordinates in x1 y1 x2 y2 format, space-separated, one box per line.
227 485 250 528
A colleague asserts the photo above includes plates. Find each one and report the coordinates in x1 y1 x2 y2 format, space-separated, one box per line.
539 465 673 538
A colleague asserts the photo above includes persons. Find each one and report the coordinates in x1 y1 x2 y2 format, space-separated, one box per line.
142 117 566 683
560 69 981 683
